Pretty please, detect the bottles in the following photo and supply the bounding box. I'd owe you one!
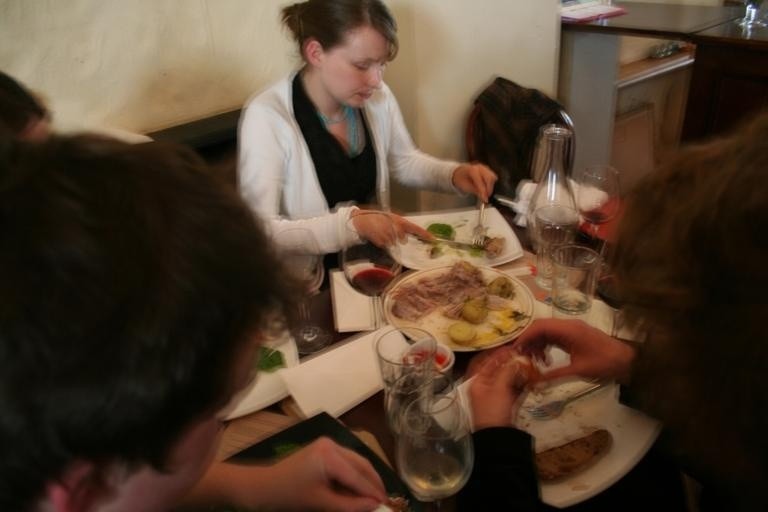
[525,127,580,253]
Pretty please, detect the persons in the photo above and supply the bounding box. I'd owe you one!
[0,125,403,512]
[442,98,768,512]
[1,72,54,143]
[235,0,499,299]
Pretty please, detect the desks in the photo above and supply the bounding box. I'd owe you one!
[560,3,765,44]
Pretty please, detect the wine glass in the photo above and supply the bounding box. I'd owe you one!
[269,228,333,356]
[376,326,475,512]
[576,163,622,238]
[342,213,402,330]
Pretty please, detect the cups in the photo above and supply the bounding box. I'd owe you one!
[534,206,603,313]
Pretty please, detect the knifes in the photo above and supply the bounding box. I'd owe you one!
[426,236,484,251]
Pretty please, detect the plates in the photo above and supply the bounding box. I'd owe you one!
[220,336,300,425]
[380,260,536,354]
[424,371,663,507]
[381,205,524,270]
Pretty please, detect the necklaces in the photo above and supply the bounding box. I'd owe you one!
[317,107,358,159]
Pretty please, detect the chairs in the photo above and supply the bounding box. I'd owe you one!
[466,75,577,208]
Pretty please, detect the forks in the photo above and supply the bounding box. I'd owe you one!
[472,199,487,247]
[527,382,611,420]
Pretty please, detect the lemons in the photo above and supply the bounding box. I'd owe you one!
[446,320,475,344]
